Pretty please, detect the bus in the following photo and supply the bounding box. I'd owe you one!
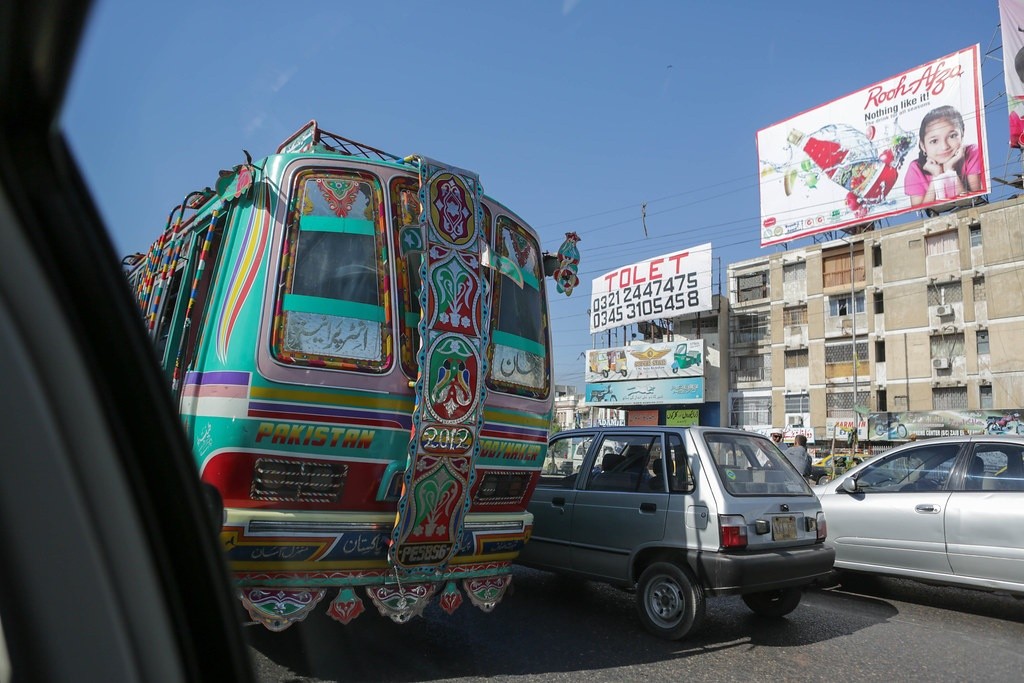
[128,126,582,606]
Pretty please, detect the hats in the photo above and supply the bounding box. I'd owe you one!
[770,428,782,434]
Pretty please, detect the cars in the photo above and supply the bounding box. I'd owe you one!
[514,426,837,643]
[815,452,888,487]
[808,436,1023,599]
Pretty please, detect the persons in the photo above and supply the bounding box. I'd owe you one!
[592,444,653,481]
[904,105,981,205]
[756,430,788,467]
[1010,46,1024,147]
[782,435,812,483]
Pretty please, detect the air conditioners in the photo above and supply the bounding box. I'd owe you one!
[789,415,801,426]
[935,304,953,318]
[932,358,949,370]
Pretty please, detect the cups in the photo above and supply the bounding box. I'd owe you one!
[934,174,955,200]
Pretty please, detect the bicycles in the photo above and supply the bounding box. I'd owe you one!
[874,415,908,438]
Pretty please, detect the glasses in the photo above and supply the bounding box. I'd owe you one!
[771,434,782,438]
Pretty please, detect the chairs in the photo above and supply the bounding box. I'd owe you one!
[653,459,676,490]
[593,453,637,489]
[981,471,1002,490]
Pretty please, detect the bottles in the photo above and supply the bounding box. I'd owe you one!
[787,130,898,204]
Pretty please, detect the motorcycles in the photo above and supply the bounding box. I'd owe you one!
[670,343,704,373]
[587,352,610,377]
[984,410,1024,434]
[607,349,629,377]
